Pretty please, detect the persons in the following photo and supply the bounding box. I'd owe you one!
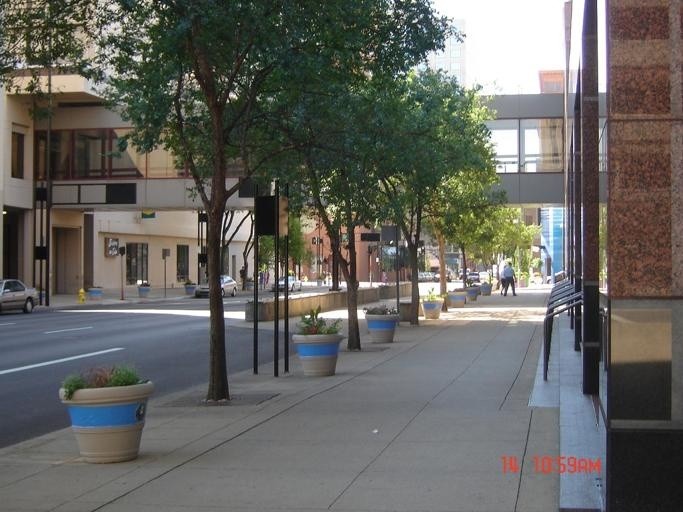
[504,263,516,296]
[501,265,507,295]
[240,266,246,280]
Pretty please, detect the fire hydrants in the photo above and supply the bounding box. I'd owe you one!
[78,288,86,304]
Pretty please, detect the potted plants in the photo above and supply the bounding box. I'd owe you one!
[291,281,494,378]
[58,363,155,464]
[87,278,199,301]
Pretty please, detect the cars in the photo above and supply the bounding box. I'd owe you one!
[195,275,238,298]
[407,270,452,282]
[272,276,302,292]
[0,279,38,314]
[458,268,491,282]
